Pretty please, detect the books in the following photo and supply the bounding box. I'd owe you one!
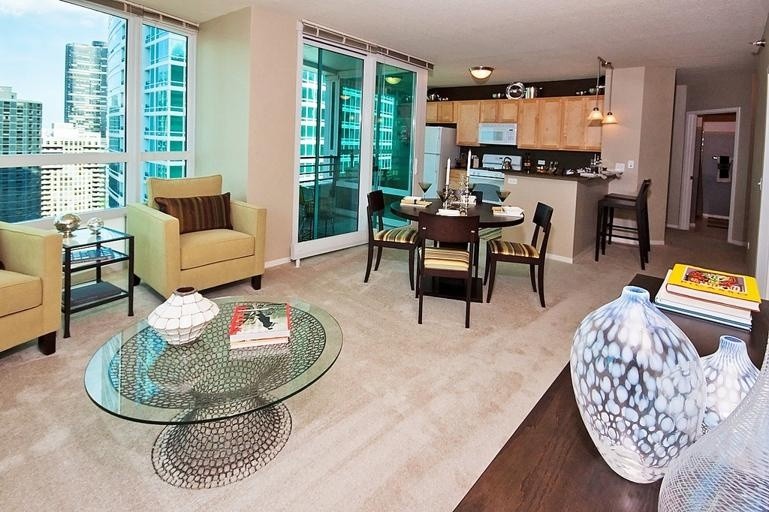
[652,262,762,331]
[491,206,525,218]
[227,302,292,349]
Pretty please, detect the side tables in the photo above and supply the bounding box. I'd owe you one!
[57,225,135,338]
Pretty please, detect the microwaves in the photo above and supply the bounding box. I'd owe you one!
[477,122,517,145]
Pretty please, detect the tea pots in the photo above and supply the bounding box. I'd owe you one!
[498,157,512,170]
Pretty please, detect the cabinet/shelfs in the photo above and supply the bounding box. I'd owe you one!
[517,97,561,150]
[426,101,453,123]
[481,99,518,123]
[561,95,604,152]
[455,100,480,147]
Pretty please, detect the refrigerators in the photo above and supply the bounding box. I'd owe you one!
[420,126,460,198]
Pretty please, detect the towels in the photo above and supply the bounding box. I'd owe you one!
[401,199,432,205]
[492,207,512,211]
[460,208,466,216]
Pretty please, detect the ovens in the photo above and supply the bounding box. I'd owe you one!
[467,176,504,206]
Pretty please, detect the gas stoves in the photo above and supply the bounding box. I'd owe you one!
[469,153,522,179]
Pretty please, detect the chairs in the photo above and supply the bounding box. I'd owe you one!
[300,185,335,239]
[483,202,553,308]
[595,183,650,271]
[416,210,480,329]
[434,187,483,278]
[604,178,650,250]
[364,190,419,290]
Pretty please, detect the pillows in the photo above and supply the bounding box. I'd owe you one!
[155,192,233,235]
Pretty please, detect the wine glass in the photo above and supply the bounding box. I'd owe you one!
[437,191,449,210]
[420,183,432,200]
[465,182,476,195]
[495,191,511,214]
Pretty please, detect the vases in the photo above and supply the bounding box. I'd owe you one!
[700,334,761,439]
[570,285,707,485]
[657,326,769,512]
[146,286,220,346]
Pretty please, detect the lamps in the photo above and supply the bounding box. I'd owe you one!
[587,57,606,120]
[601,61,618,123]
[385,77,401,85]
[341,95,350,100]
[469,66,493,79]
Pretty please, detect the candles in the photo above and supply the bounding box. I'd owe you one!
[467,149,471,176]
[446,157,451,184]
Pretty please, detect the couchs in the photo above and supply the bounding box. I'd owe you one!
[0,221,63,355]
[127,178,267,300]
